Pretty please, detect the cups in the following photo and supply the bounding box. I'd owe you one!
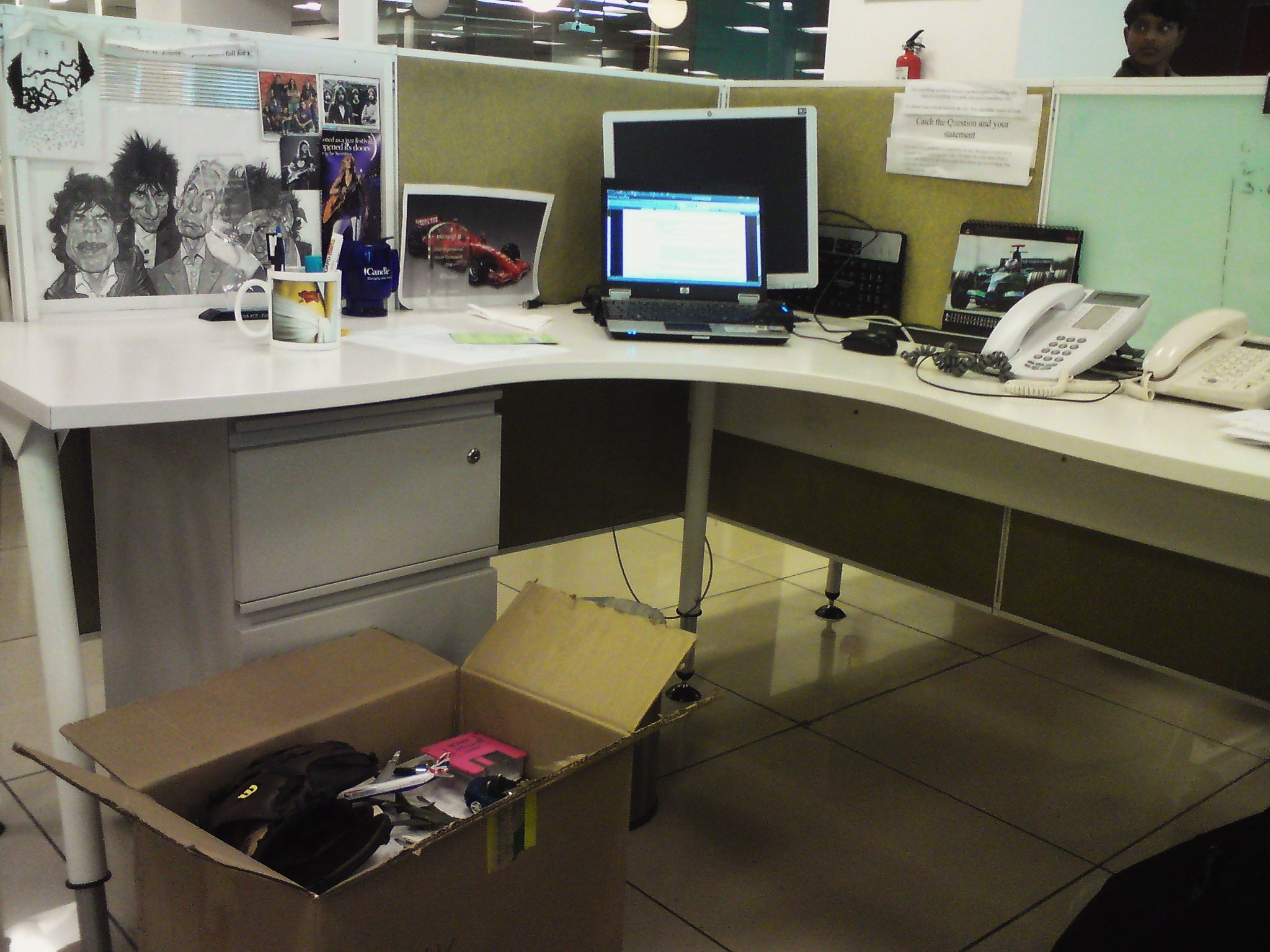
[334,242,393,318]
[235,266,342,351]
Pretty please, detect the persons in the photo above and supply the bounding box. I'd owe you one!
[288,140,314,190]
[329,154,369,241]
[262,74,319,137]
[323,85,377,126]
[1112,0,1187,78]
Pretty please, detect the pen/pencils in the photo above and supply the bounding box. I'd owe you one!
[274,225,285,271]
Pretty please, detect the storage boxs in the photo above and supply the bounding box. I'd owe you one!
[14,584,700,952]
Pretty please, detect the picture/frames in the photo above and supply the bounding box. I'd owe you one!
[257,69,321,142]
[319,74,383,135]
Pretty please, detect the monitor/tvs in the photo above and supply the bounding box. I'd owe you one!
[604,105,819,290]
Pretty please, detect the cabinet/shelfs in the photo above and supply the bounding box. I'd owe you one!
[89,385,504,712]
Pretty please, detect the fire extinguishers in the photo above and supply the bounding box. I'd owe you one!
[895,28,926,80]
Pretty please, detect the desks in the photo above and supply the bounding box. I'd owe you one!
[0,298,1269,891]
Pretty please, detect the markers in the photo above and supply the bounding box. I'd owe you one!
[324,233,343,273]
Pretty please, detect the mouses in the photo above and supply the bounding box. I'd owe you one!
[840,330,899,356]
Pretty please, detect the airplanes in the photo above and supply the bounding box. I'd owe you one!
[337,750,461,831]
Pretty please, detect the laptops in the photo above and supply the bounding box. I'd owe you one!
[600,176,792,343]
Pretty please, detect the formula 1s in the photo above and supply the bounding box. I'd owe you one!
[406,215,531,285]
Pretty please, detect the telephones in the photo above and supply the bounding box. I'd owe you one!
[976,282,1154,380]
[1139,307,1270,411]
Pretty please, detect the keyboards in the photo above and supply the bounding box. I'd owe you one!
[768,224,908,322]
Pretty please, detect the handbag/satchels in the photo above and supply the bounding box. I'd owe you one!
[189,737,393,894]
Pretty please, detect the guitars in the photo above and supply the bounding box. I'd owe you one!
[286,164,319,185]
[323,168,377,225]
